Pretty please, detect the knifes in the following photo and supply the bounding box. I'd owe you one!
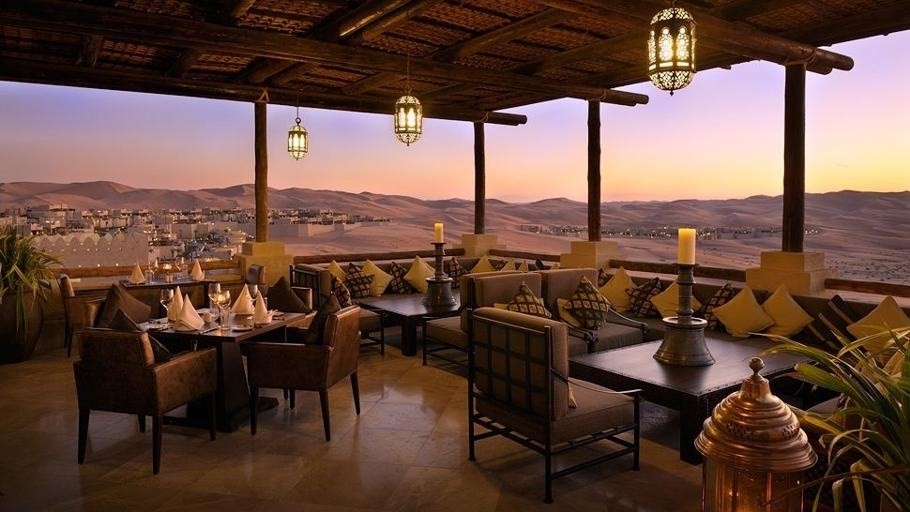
[200,325,220,334]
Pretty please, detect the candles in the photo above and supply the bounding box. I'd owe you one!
[434,222,445,242]
[678,226,697,264]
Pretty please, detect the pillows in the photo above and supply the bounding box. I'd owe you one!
[94,282,154,326]
[264,276,313,315]
[109,310,173,363]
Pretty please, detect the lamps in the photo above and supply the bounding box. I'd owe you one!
[394,44,425,147]
[286,79,309,162]
[644,0,697,97]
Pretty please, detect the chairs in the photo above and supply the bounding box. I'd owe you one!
[243,263,267,296]
[69,326,220,475]
[238,284,317,359]
[243,303,364,442]
[289,256,597,376]
[468,266,890,507]
[82,296,108,328]
[58,273,87,358]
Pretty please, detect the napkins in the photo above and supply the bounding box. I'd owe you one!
[175,293,205,332]
[168,286,184,321]
[253,288,277,324]
[190,259,205,281]
[230,282,254,313]
[128,260,146,284]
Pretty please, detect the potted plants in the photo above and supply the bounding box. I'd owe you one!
[1,220,72,364]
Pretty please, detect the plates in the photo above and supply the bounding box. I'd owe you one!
[145,323,163,329]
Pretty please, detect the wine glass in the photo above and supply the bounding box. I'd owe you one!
[160,282,267,330]
[144,256,189,283]
